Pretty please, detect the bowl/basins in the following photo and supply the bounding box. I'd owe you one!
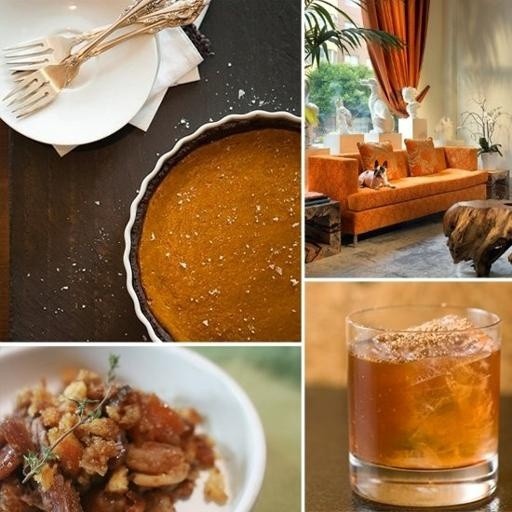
[122,110,302,343]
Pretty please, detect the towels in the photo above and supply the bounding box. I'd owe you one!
[51,0,212,158]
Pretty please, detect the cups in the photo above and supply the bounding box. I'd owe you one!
[345,303,503,511]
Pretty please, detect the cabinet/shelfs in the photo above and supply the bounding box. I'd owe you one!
[480,168,512,199]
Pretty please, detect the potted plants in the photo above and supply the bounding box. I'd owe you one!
[459,98,512,170]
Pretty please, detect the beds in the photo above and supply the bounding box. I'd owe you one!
[305,200,341,263]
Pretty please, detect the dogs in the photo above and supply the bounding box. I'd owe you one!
[357,159,396,191]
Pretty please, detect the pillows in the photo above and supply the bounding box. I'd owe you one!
[357,141,400,181]
[404,137,439,177]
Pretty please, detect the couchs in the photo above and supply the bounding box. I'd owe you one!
[308,146,489,243]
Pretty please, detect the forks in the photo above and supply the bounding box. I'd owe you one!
[1,0,211,118]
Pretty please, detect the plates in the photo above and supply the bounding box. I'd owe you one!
[1,346,268,511]
[0,0,159,146]
